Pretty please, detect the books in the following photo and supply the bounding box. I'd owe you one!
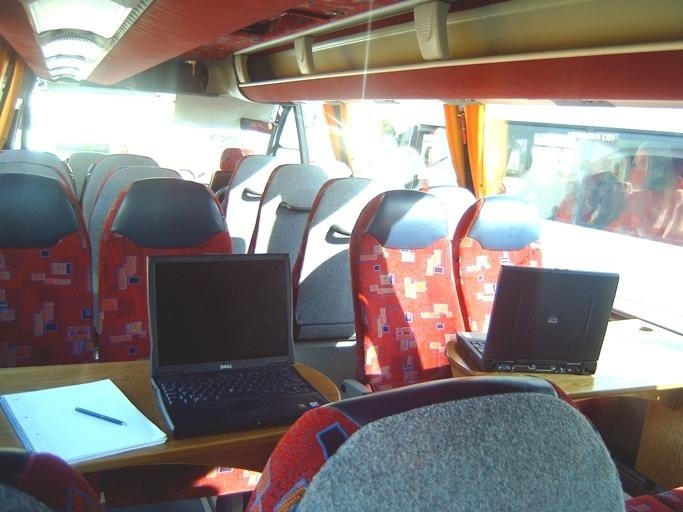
[0,377,168,467]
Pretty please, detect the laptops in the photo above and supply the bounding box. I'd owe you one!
[146,253,331,438]
[456,265,619,374]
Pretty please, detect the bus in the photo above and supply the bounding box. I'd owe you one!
[306,96,683,361]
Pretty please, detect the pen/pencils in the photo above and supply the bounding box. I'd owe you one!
[75,407,126,425]
